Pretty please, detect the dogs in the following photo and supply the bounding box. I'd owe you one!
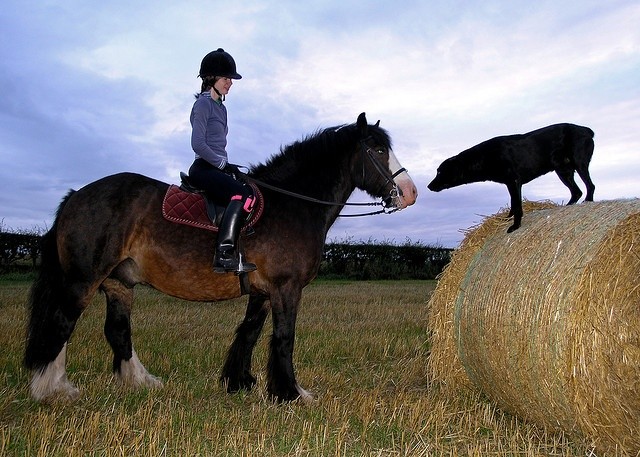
[426,120,596,233]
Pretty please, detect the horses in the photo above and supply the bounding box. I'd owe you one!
[20,111,420,411]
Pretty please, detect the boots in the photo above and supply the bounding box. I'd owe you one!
[212,198,257,273]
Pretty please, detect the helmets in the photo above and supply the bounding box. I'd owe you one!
[200,48,242,80]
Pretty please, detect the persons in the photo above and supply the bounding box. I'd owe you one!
[188,48,260,274]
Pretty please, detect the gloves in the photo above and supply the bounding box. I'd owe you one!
[224,163,239,176]
